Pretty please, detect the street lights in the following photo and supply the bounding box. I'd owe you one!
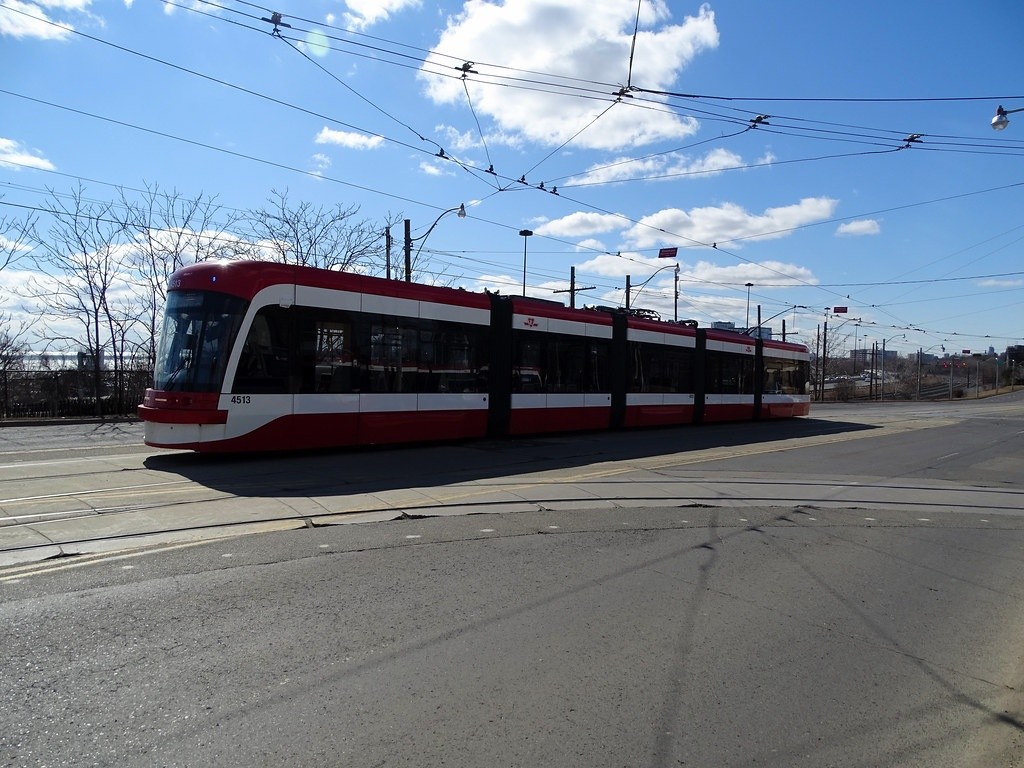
[744,304,807,338]
[401,202,467,283]
[816,318,862,402]
[915,344,947,400]
[879,334,907,401]
[947,350,1024,401]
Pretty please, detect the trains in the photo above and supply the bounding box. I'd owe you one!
[139,260,810,456]
